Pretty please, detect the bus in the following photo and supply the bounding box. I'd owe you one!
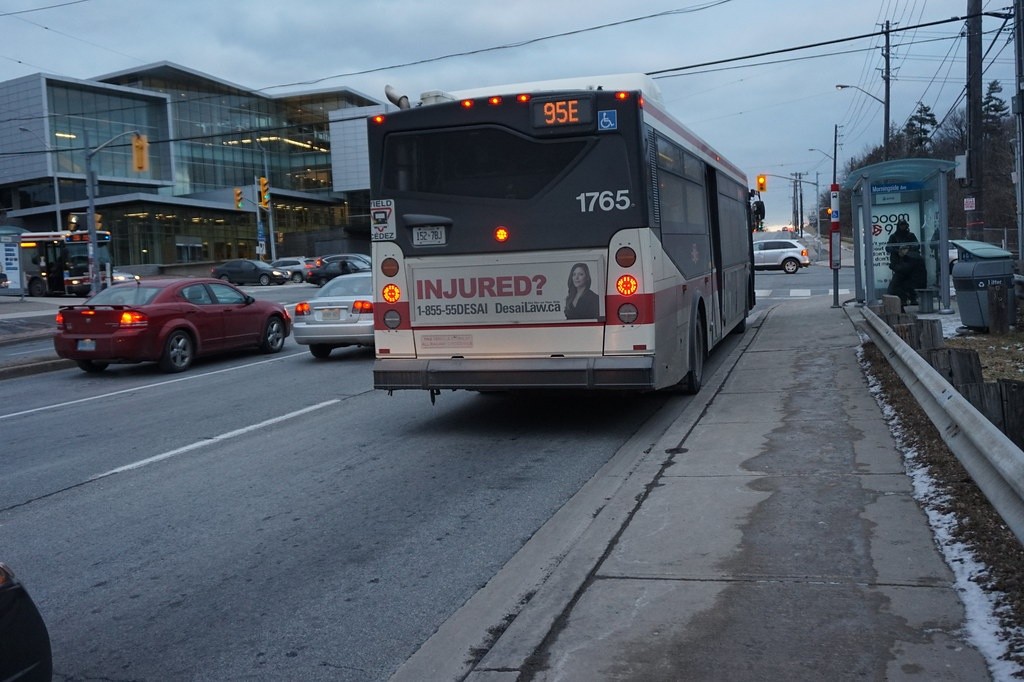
[21,230,112,296]
[366,73,766,396]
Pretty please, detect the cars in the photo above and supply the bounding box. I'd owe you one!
[111,269,139,285]
[211,258,291,285]
[946,228,969,274]
[53,274,291,373]
[292,272,375,357]
[782,227,788,231]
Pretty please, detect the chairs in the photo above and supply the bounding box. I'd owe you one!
[187,287,204,304]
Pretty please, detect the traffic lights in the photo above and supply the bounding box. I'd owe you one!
[756,175,765,191]
[234,188,243,210]
[827,208,831,219]
[95,213,103,231]
[68,212,88,232]
[260,177,270,209]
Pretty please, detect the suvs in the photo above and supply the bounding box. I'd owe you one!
[269,253,373,288]
[753,239,810,274]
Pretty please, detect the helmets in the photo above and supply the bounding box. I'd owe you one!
[896,219,909,229]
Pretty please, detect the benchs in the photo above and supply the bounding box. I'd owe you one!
[915,288,939,313]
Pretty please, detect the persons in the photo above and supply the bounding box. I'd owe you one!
[564,264,598,320]
[0,262,8,289]
[885,220,928,313]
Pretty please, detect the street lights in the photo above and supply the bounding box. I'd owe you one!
[836,19,890,161]
[809,124,844,185]
[18,127,62,232]
[235,125,276,262]
